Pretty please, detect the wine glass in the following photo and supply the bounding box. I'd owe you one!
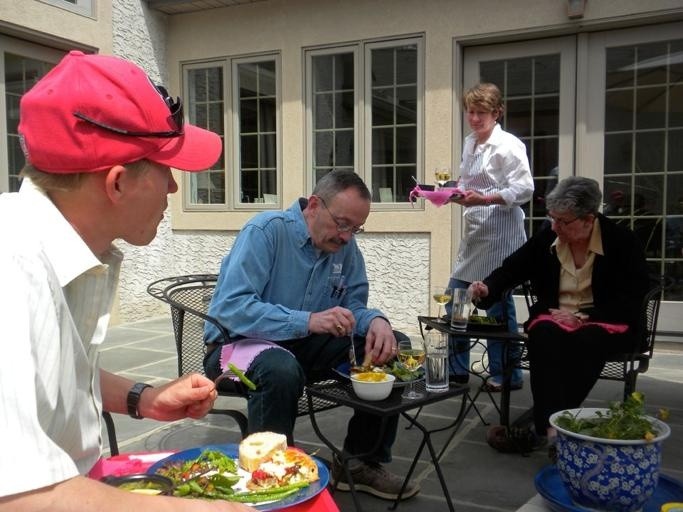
[430,287,450,325]
[397,341,425,399]
[435,168,449,187]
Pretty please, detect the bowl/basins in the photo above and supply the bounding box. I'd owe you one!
[418,184,433,190]
[440,181,457,188]
[351,372,396,401]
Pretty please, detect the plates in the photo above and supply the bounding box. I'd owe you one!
[101,473,172,495]
[442,316,506,332]
[334,361,427,388]
[145,442,329,511]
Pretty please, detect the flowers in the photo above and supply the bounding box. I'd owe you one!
[546,385,670,448]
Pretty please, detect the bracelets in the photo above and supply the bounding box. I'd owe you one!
[127,383,152,419]
[575,316,585,324]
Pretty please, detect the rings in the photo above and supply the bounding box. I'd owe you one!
[391,347,398,352]
[337,324,342,331]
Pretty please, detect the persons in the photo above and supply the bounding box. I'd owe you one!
[202,170,421,502]
[466,176,649,465]
[0,48,263,512]
[444,82,535,392]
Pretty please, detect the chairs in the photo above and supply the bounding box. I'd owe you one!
[98,401,250,499]
[143,271,352,459]
[490,264,675,437]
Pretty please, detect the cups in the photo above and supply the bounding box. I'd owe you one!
[449,287,472,333]
[424,332,448,392]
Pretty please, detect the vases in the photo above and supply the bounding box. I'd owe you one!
[544,408,672,511]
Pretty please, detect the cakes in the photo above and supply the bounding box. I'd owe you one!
[237,430,288,473]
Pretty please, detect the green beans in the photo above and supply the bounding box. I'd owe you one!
[225,363,257,392]
[212,483,310,503]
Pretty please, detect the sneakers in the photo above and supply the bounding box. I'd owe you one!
[479,379,524,393]
[486,419,551,454]
[329,448,421,500]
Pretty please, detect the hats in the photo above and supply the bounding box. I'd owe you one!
[20,50,222,172]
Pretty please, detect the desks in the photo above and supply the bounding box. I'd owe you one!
[299,364,471,511]
[398,312,529,433]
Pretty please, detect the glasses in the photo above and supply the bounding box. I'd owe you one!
[317,195,365,235]
[72,85,186,138]
[546,214,581,227]
